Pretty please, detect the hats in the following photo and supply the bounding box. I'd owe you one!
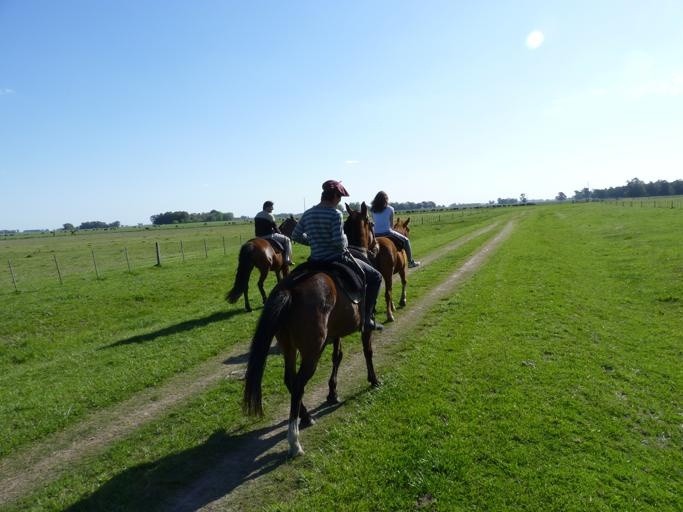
[322,180,350,197]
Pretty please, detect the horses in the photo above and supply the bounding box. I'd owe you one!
[242,200,382,459]
[226,213,299,313]
[375,216,413,323]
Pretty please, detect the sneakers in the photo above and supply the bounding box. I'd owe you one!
[369,319,384,330]
[285,261,295,265]
[408,261,420,268]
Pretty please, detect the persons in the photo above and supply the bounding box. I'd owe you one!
[253,201,296,266]
[368,191,421,268]
[291,180,384,331]
